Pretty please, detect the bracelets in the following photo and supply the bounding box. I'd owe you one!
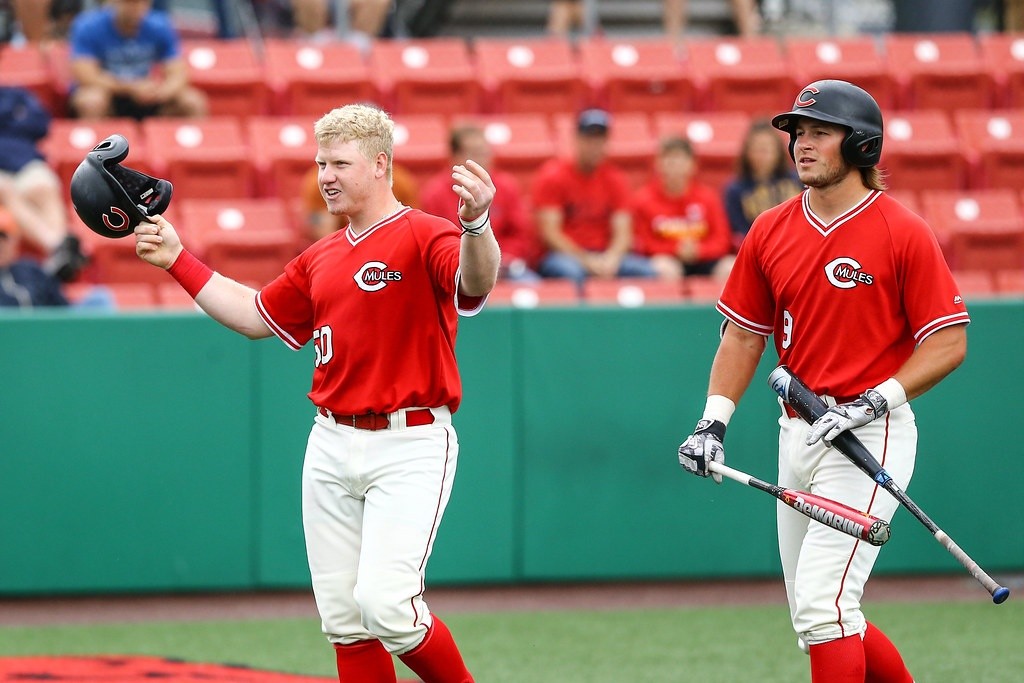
[459,213,490,236]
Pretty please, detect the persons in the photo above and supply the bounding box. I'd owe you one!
[722,116,803,255]
[419,122,538,283]
[1,0,978,46]
[133,103,502,683]
[66,0,213,124]
[629,132,739,281]
[678,79,971,683]
[0,85,88,279]
[515,108,658,280]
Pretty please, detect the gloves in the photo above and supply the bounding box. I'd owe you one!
[805,388,888,448]
[678,419,727,484]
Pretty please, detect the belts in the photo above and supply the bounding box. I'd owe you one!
[783,395,860,419]
[320,406,434,431]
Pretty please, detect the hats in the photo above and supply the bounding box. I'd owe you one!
[576,106,612,135]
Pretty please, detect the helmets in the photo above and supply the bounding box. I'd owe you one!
[771,80,883,169]
[69,134,174,239]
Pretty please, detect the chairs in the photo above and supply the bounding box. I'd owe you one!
[2,33,1024,309]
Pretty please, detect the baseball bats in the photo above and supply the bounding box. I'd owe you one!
[707,460,892,547]
[765,362,1011,606]
